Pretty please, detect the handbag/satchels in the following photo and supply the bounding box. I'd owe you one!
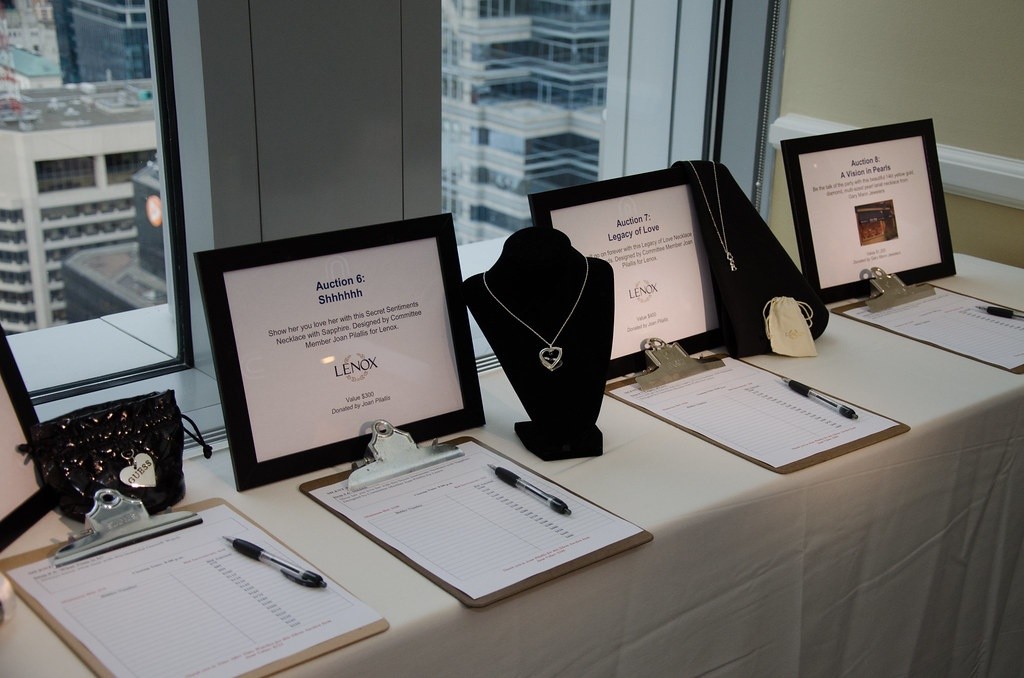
[15,388,214,524]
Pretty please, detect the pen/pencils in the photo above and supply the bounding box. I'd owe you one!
[975,305,1024,320]
[487,464,572,516]
[782,377,858,419]
[221,535,327,588]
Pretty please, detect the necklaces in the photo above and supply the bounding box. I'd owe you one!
[688,161,737,271]
[483,256,589,372]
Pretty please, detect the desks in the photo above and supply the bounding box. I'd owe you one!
[1,250,1024,677]
